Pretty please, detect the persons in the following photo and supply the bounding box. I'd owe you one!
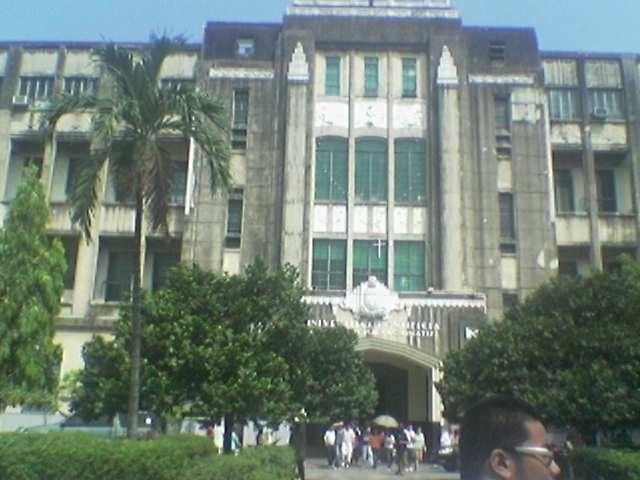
[207,425,214,440]
[323,419,427,476]
[231,429,241,456]
[459,394,561,480]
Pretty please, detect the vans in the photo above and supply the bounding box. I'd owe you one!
[16,408,160,439]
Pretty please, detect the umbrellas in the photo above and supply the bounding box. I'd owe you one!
[372,414,400,430]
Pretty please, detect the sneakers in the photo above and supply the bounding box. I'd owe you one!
[328,460,418,477]
